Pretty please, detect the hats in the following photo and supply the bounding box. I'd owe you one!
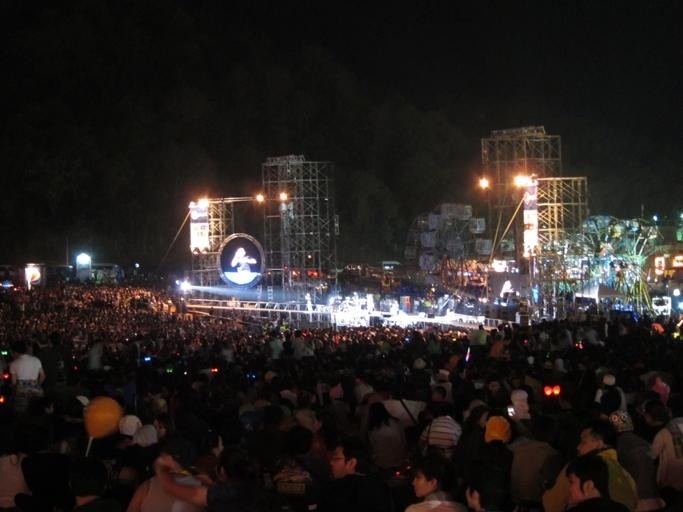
[117,414,142,437]
[129,423,160,447]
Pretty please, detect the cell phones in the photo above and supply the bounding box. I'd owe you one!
[507,406,515,418]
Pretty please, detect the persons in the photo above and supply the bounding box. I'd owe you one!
[1,267,683,510]
